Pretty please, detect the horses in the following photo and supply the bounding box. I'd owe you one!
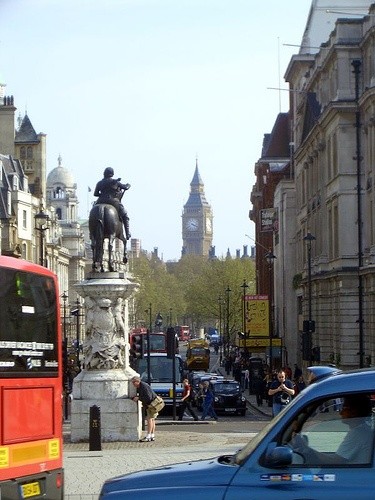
[88,188,127,272]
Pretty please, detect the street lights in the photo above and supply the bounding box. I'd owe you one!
[70,298,82,373]
[145,302,152,331]
[61,290,69,391]
[32,204,54,267]
[217,296,223,364]
[225,284,232,354]
[169,307,173,327]
[303,232,317,368]
[239,279,250,360]
[264,250,277,372]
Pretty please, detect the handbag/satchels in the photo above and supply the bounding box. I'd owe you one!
[281,393,290,404]
[149,396,166,412]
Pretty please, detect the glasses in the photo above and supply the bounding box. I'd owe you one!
[279,376,285,378]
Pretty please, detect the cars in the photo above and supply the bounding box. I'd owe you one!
[185,370,248,416]
[101,366,375,499]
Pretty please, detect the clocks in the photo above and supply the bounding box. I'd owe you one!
[186,217,200,232]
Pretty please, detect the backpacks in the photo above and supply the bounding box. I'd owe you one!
[189,386,196,399]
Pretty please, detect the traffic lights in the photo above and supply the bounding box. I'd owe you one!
[132,334,144,359]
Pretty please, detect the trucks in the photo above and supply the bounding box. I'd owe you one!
[186,338,211,371]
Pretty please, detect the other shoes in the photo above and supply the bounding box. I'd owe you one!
[139,436,151,442]
[151,436,156,441]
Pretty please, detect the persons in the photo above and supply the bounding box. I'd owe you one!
[197,381,218,420]
[93,167,132,240]
[268,371,295,418]
[131,376,159,442]
[216,339,346,411]
[287,391,374,465]
[177,379,199,421]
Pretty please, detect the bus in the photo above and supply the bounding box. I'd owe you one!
[130,328,181,355]
[0,258,67,500]
[176,325,191,341]
[136,353,188,416]
